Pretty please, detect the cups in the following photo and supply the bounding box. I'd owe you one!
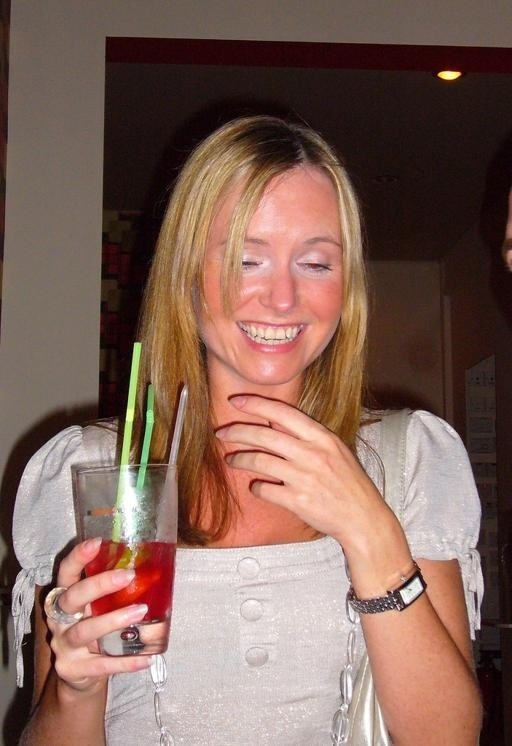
[71,464,179,659]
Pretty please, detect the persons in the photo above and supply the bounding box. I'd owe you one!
[11,112,492,745]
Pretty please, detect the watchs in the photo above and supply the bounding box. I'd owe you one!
[346,559,431,615]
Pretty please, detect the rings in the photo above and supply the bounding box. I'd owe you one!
[45,585,86,626]
